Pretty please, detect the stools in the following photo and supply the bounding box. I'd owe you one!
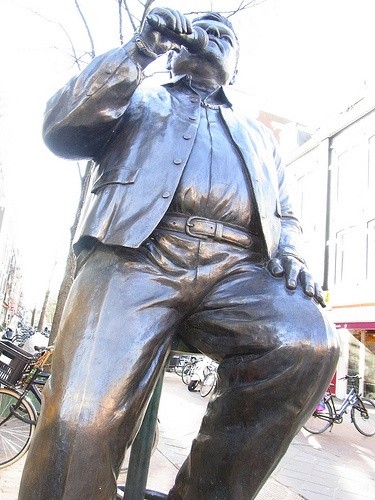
[115,332,201,500]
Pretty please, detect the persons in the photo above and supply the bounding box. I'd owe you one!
[17,5,340,500]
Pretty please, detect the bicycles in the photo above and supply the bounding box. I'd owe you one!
[302,375,375,437]
[174,355,221,397]
[0,339,160,474]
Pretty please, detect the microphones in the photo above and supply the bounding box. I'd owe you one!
[146,14,209,54]
